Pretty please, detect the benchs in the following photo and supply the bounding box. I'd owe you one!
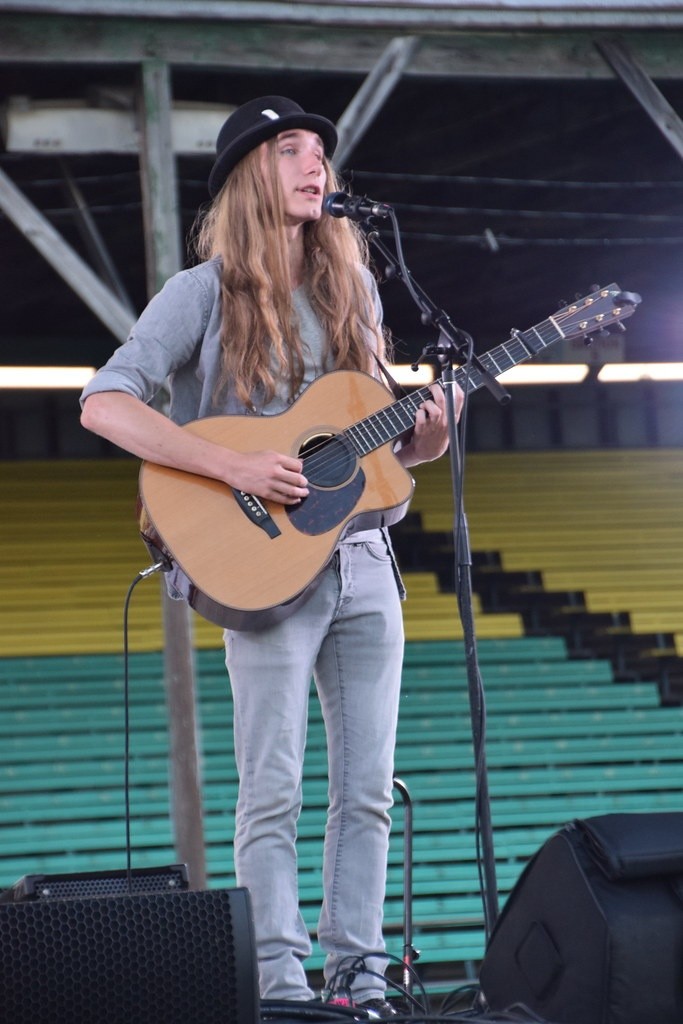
[0,442,682,1005]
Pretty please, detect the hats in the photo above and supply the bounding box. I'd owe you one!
[210,94,340,196]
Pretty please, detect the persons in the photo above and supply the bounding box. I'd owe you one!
[74,94,466,1013]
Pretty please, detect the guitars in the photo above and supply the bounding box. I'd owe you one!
[140,281,642,632]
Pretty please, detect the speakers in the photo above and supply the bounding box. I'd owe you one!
[0,886,265,1024]
[479,810,683,1024]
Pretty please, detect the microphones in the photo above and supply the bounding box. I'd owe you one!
[325,191,393,219]
